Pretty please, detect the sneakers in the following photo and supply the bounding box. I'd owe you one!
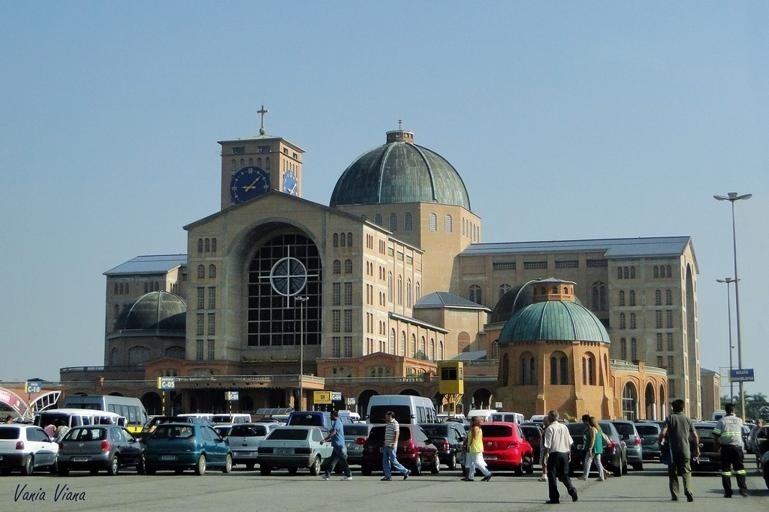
[321,470,752,505]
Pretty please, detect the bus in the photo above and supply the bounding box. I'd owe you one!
[363,394,439,424]
[63,395,149,426]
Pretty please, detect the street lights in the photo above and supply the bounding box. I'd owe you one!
[292,294,312,411]
[712,189,754,422]
[716,276,744,415]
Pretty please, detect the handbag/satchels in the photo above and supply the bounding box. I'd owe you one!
[660,418,674,466]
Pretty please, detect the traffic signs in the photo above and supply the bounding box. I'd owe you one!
[729,369,754,382]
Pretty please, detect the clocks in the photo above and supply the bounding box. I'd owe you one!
[230,166,270,206]
[282,170,299,198]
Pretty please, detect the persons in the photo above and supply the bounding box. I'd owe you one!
[712,403,751,499]
[461,410,613,505]
[749,418,764,472]
[0,415,112,443]
[658,399,699,502]
[320,410,352,481]
[380,411,411,481]
